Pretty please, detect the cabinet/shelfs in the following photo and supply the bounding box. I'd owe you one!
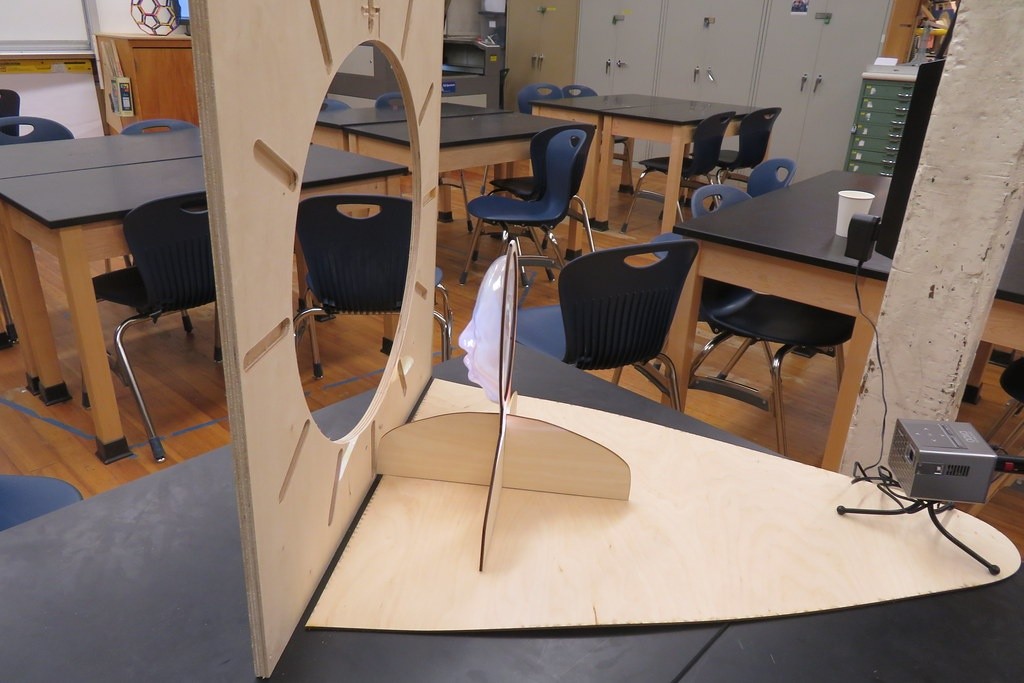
[502,0,920,190]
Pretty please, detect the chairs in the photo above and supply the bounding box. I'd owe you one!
[374,91,473,233]
[120,119,196,334]
[296,194,453,377]
[981,356,1024,453]
[459,83,882,460]
[320,99,351,114]
[0,89,20,136]
[82,190,224,462]
[0,116,74,338]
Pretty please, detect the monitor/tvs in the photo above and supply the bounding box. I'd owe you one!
[173,0,189,25]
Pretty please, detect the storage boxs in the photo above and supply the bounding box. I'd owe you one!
[110,76,134,117]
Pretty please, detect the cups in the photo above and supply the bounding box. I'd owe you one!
[835,189,875,238]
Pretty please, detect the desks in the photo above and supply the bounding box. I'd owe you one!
[593,102,764,236]
[676,479,1024,683]
[0,336,794,683]
[0,128,317,396]
[0,143,409,465]
[971,299,1024,406]
[661,170,892,471]
[312,101,513,224]
[528,94,683,229]
[342,112,597,262]
